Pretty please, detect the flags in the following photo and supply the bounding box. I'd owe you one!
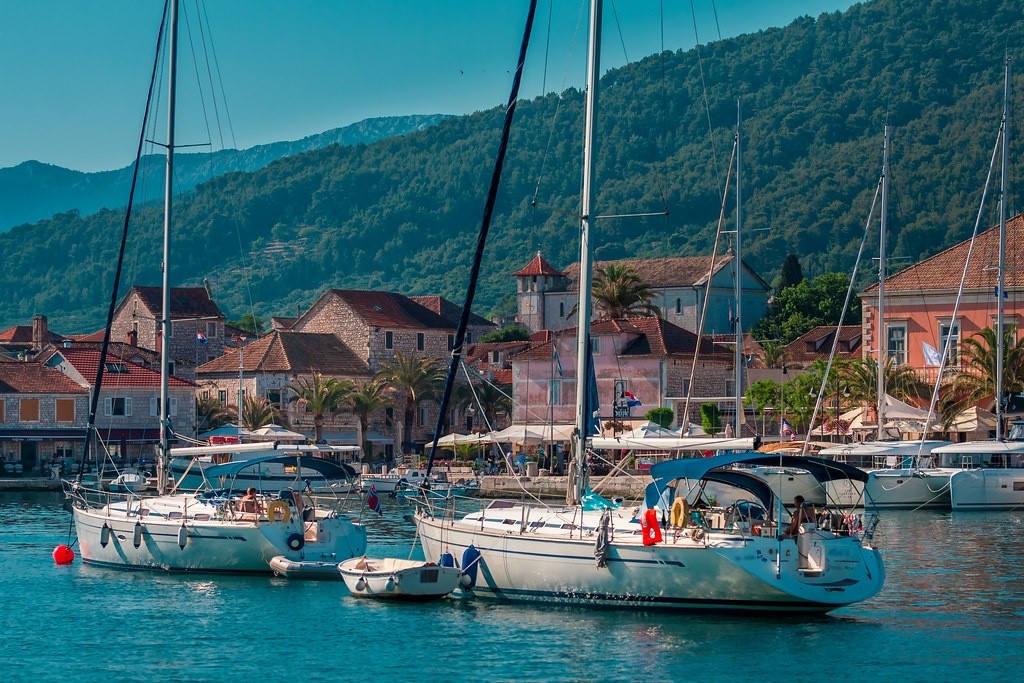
[922,341,942,366]
[779,418,796,442]
[368,485,383,517]
[553,341,563,376]
[197,334,209,344]
[624,391,641,407]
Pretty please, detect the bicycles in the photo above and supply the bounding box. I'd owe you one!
[30,463,56,481]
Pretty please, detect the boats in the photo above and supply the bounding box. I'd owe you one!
[337,556,464,601]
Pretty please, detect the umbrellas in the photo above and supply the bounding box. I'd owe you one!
[424,429,545,460]
[242,423,305,441]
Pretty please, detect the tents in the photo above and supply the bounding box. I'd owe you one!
[810,393,996,435]
[495,424,576,458]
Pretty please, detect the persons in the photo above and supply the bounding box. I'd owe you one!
[111,451,123,464]
[240,486,261,514]
[783,495,813,545]
[0,451,16,462]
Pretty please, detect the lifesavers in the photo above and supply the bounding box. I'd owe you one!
[668,496,689,528]
[267,499,291,525]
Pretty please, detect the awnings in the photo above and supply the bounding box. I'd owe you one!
[617,419,707,438]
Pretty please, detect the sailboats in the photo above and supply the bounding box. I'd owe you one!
[656,40,1023,512]
[398,0,890,614]
[60,0,385,579]
